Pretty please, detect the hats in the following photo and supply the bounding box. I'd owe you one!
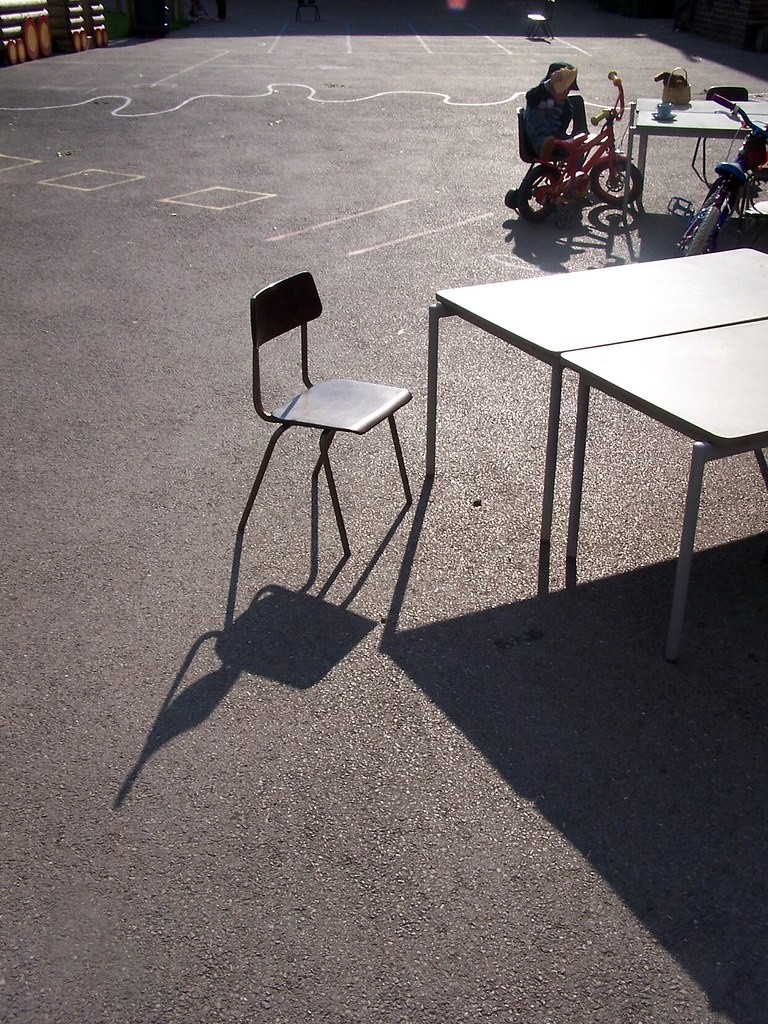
[543,62,579,91]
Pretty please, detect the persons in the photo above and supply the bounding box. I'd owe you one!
[521,61,596,206]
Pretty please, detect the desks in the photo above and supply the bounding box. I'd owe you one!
[424,248,768,544]
[559,319,768,661]
[621,98,768,218]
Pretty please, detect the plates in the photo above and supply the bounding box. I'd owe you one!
[652,113,678,120]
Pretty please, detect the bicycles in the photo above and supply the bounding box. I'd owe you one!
[668,89,768,257]
[505,71,643,222]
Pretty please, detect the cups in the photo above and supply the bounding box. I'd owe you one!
[651,103,677,121]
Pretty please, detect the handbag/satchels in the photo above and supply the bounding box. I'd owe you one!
[662,67,691,105]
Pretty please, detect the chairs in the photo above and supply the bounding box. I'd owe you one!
[566,95,589,140]
[239,270,413,557]
[526,0,555,40]
[691,85,748,177]
[516,106,564,190]
[296,0,320,22]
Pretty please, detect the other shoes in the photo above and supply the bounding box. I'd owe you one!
[578,196,594,207]
